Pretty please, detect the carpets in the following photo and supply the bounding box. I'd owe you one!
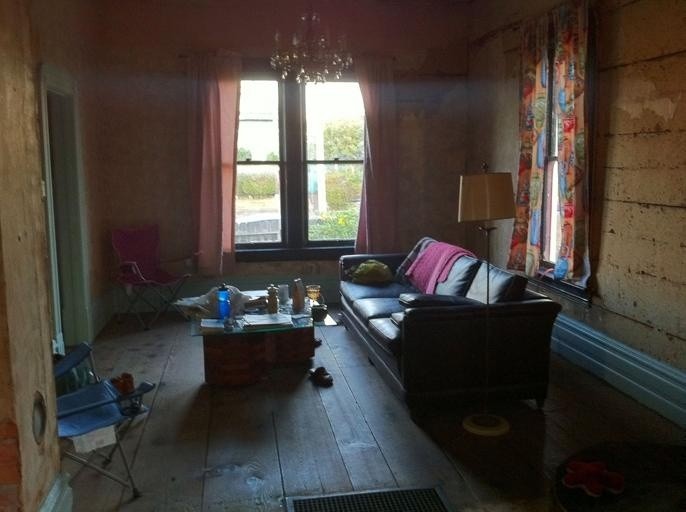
[284,485,457,512]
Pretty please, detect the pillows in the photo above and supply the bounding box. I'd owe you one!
[434,255,481,297]
[463,262,528,304]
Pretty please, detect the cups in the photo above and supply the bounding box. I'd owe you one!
[311,304,328,321]
[277,284,288,302]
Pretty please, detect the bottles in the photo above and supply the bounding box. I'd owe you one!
[215,282,231,320]
[266,283,279,314]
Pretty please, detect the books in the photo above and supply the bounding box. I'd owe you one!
[236,311,295,332]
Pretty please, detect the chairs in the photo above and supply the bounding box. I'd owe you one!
[109,223,202,331]
[54,341,156,498]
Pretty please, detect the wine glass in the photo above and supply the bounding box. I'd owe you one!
[306,284,320,306]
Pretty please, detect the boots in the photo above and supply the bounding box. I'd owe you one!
[111,373,135,394]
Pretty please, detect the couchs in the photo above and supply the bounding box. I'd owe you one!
[338,237,562,418]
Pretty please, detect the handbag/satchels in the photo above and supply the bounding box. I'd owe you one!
[352,262,393,283]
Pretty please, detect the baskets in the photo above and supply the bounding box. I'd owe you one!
[311,293,328,322]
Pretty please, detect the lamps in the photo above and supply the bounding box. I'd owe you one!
[458,159,517,437]
[270,0,353,84]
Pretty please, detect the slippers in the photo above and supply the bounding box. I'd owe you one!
[308,366,333,385]
